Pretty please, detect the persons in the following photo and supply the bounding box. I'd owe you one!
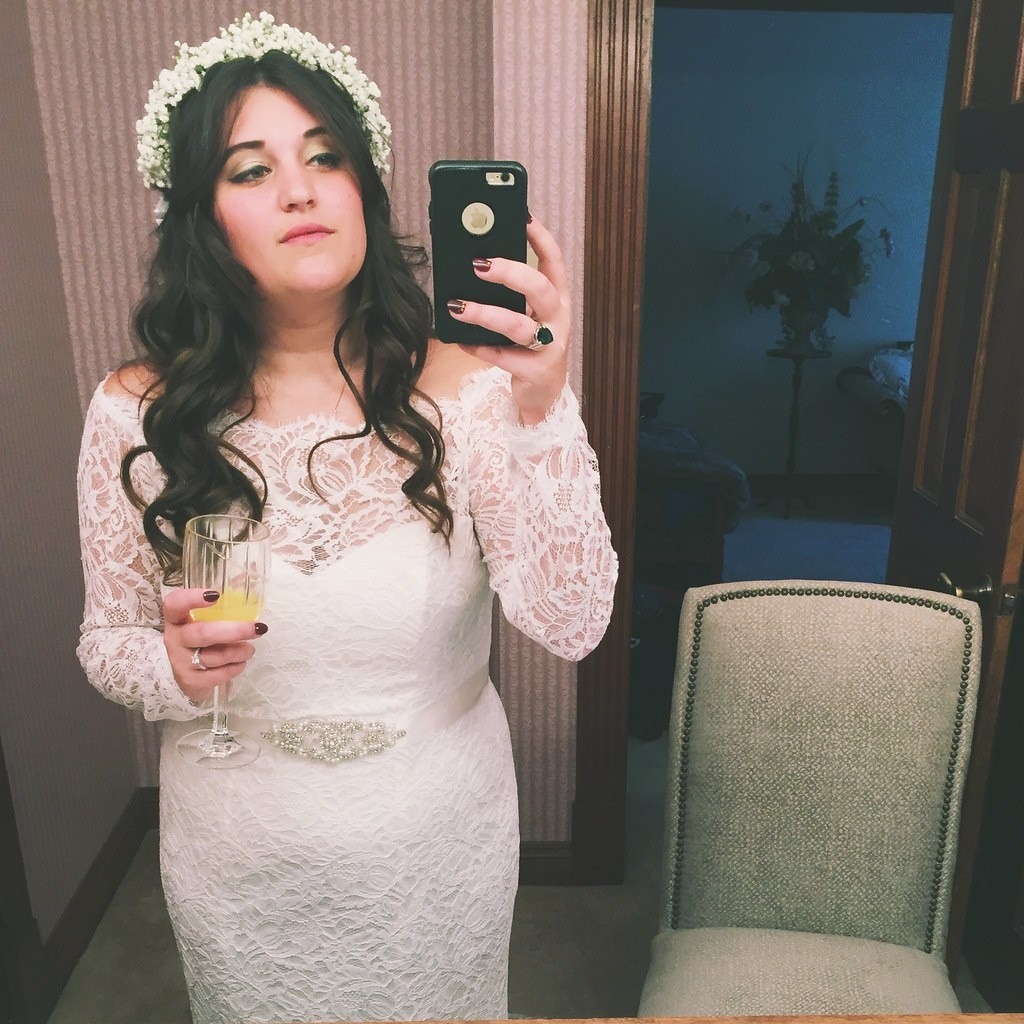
[74,10,619,1022]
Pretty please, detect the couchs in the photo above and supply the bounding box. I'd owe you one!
[627,390,754,638]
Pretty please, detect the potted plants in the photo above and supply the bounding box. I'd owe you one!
[743,147,895,519]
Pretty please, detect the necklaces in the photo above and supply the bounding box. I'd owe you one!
[261,341,355,427]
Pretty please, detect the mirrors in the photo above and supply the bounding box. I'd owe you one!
[0,0,1023,1024]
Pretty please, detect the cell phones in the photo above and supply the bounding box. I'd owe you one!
[428,160,527,344]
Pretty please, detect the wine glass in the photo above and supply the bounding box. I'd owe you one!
[178,515,272,771]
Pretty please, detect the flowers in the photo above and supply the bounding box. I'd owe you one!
[136,11,393,192]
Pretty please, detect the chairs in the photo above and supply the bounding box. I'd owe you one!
[634,576,983,1018]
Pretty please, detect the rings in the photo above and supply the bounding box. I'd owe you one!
[190,648,206,671]
[526,320,553,352]
